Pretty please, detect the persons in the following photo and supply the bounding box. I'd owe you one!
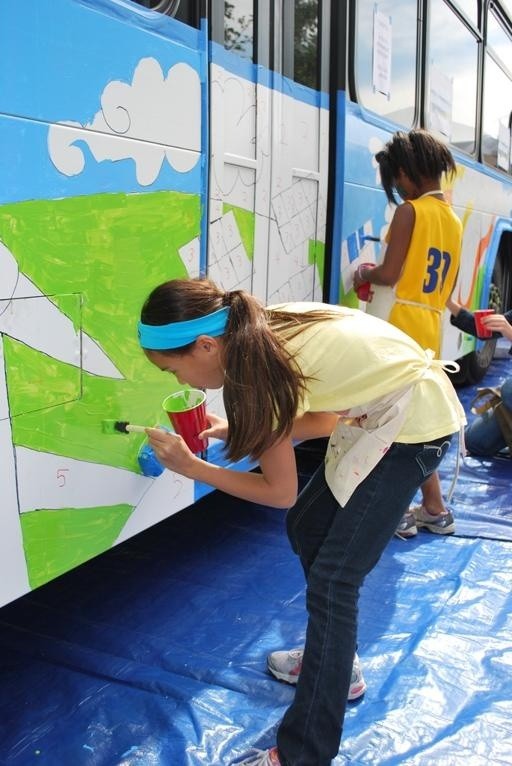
[138,277,467,766]
[354,128,462,542]
[445,293,512,457]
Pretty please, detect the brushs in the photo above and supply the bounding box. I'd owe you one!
[102,420,182,441]
[362,235,380,241]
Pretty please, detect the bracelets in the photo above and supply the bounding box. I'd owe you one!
[358,264,366,279]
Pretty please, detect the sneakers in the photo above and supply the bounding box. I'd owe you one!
[232,745,282,766]
[264,647,367,703]
[408,504,456,536]
[394,512,418,539]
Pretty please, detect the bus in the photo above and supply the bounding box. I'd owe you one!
[0,0,511,613]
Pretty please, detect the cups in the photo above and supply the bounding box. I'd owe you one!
[357,262,376,301]
[473,309,496,339]
[161,389,212,454]
